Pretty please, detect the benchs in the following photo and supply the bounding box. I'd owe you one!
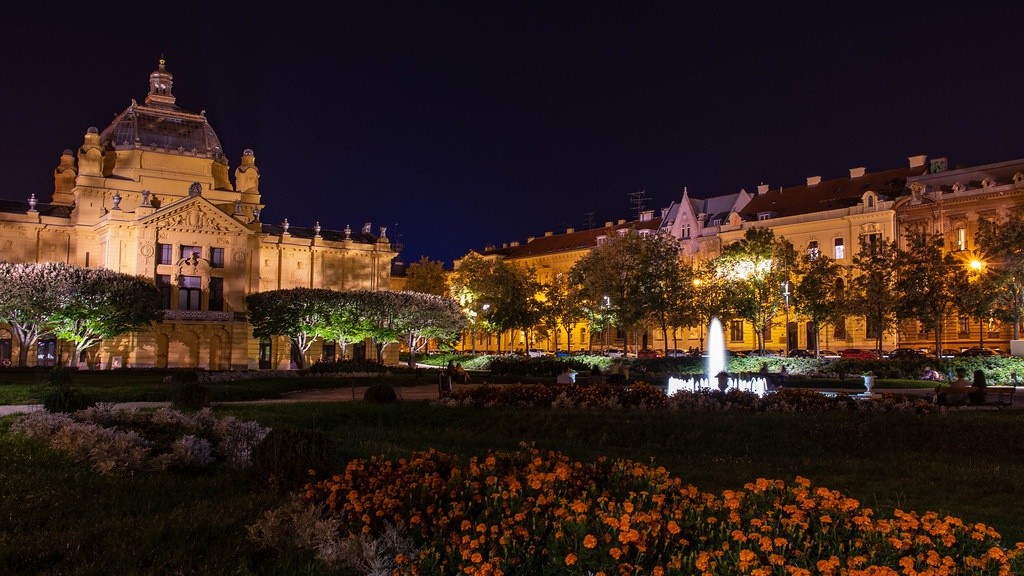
[925,385,1017,407]
[442,371,491,385]
[555,372,652,386]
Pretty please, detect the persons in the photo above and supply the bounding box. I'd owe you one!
[940,368,987,405]
[448,360,472,384]
